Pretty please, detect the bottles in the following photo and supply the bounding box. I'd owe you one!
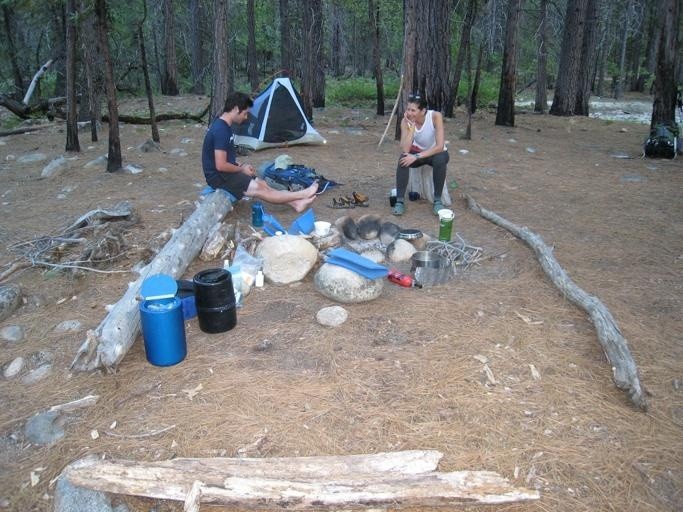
[388,270,423,290]
[256,271,264,286]
[252,200,265,226]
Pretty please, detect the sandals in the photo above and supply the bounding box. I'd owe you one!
[348,192,369,207]
[326,195,355,208]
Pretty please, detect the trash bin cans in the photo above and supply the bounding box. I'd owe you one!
[138,272,187,366]
[193,268,237,334]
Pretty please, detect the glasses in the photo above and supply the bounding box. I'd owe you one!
[409,94,422,99]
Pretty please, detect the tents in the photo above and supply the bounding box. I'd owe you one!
[224,76,327,151]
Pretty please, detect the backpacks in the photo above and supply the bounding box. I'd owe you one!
[259,161,336,194]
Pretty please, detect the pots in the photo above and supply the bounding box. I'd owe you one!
[411,250,442,268]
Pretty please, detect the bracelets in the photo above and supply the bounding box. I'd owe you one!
[414,153,420,160]
[408,127,415,132]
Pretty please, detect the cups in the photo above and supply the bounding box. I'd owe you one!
[439,209,455,241]
[314,221,331,235]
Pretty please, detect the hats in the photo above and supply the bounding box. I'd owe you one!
[274,155,293,170]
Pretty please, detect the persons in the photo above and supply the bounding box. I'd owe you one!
[201,90,319,214]
[391,93,450,218]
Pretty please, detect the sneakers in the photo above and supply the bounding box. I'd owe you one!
[394,202,404,215]
[432,202,445,215]
